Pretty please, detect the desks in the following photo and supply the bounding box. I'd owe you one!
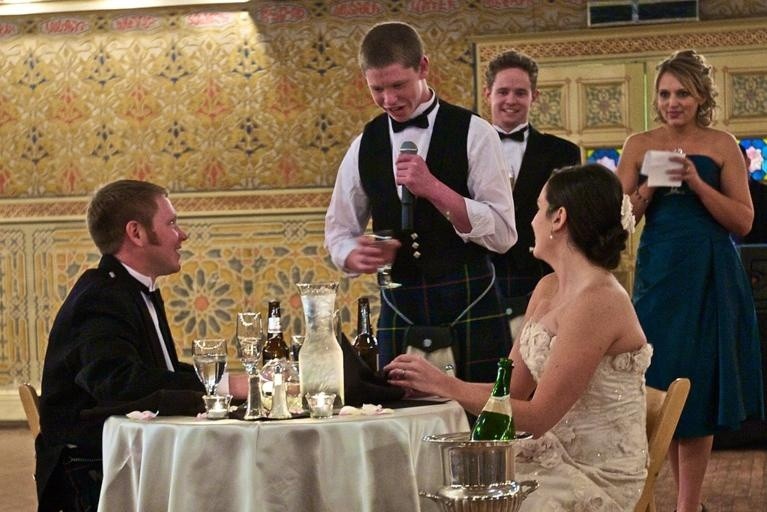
[94,394,472,512]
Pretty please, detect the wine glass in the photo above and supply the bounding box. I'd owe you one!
[192,338,228,395]
[361,227,404,290]
[236,311,264,410]
[665,148,687,197]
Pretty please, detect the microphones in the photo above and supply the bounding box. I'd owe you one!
[399,139,418,231]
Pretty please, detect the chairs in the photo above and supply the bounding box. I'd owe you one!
[631,376,691,512]
[18,383,43,439]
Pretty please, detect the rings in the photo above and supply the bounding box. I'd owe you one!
[400,370,405,378]
[685,166,689,174]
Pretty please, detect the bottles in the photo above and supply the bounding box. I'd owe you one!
[244,365,268,420]
[353,297,380,373]
[266,364,294,420]
[258,358,300,411]
[469,355,520,443]
[297,281,346,415]
[261,299,289,368]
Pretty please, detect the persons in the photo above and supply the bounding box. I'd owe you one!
[36,180,299,512]
[484,52,581,337]
[615,50,765,512]
[325,23,518,380]
[731,143,767,244]
[382,165,654,512]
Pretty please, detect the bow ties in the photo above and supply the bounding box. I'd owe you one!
[391,117,429,132]
[149,287,162,301]
[495,131,525,143]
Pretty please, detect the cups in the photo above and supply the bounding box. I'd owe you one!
[505,163,515,190]
[303,391,336,420]
[202,394,233,420]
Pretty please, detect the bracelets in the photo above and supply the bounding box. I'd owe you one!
[635,184,650,205]
